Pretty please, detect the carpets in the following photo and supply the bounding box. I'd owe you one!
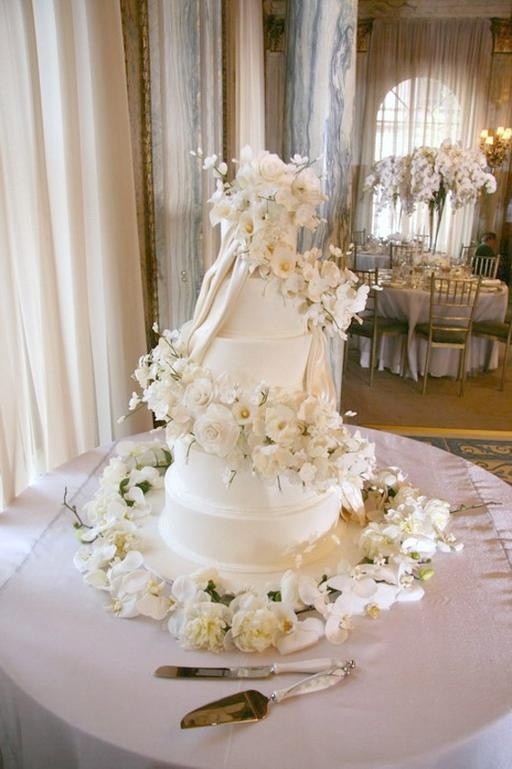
[369,426,510,492]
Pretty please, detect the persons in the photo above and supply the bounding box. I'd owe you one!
[473,232,500,278]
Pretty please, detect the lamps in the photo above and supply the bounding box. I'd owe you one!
[479,122,512,166]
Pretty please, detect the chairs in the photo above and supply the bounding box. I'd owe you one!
[340,227,512,397]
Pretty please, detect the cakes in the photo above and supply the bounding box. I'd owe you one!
[156,180,347,588]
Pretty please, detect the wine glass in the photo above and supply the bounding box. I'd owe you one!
[359,236,502,291]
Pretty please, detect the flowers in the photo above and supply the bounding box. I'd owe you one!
[360,136,497,256]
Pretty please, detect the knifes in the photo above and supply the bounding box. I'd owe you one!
[151,656,356,680]
[178,666,351,731]
